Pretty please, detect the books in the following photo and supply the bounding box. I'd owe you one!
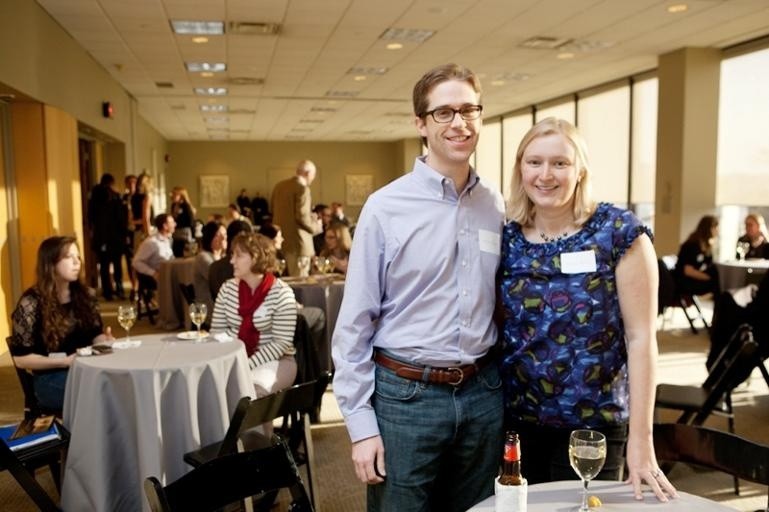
[0,413,63,453]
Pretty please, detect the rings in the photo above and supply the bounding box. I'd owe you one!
[653,469,662,478]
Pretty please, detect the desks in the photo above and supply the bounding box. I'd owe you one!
[60,332,260,511]
[713,258,767,282]
[467,476,734,510]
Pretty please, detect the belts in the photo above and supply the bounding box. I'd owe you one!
[375,353,489,384]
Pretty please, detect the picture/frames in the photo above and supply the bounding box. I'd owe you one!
[197,173,230,209]
[265,166,321,213]
[344,173,374,207]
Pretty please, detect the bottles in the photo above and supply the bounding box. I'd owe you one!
[494,429,528,511]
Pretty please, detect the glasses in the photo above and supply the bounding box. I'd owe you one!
[424,105,483,124]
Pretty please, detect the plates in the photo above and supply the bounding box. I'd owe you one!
[178,331,209,339]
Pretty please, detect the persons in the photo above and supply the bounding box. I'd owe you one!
[494,116,680,503]
[329,60,509,512]
[10,234,116,416]
[735,211,769,260]
[85,158,354,333]
[677,214,721,303]
[206,228,299,454]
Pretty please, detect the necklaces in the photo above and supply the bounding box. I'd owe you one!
[531,215,574,243]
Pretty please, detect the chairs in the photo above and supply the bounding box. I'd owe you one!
[281,313,324,428]
[5,334,70,420]
[659,253,707,333]
[622,421,768,510]
[653,321,762,497]
[714,287,768,403]
[132,247,352,338]
[182,372,331,509]
[143,433,315,509]
[1,418,73,511]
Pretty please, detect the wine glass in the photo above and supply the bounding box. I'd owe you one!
[736,242,750,263]
[277,256,336,281]
[189,303,207,342]
[118,305,136,345]
[567,428,607,512]
